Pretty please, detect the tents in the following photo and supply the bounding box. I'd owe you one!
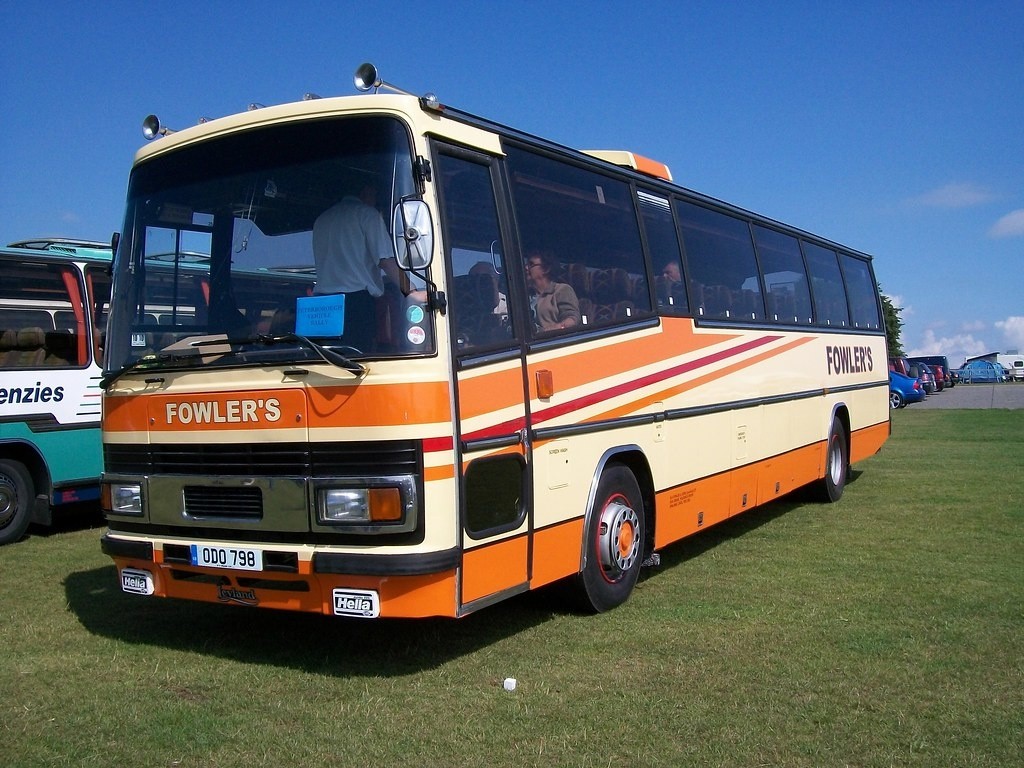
[958,361,1011,384]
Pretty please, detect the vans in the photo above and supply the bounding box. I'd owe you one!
[890,355,960,396]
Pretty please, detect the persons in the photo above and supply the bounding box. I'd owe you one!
[309,158,433,362]
[458,259,516,349]
[659,259,691,284]
[503,248,582,343]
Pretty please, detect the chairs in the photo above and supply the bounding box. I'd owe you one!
[452,257,876,351]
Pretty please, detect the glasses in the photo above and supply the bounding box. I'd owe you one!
[525,263,543,267]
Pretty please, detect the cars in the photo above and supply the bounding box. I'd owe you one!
[888,368,925,409]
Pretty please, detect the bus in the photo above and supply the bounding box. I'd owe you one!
[0,238,320,551]
[95,64,889,625]
